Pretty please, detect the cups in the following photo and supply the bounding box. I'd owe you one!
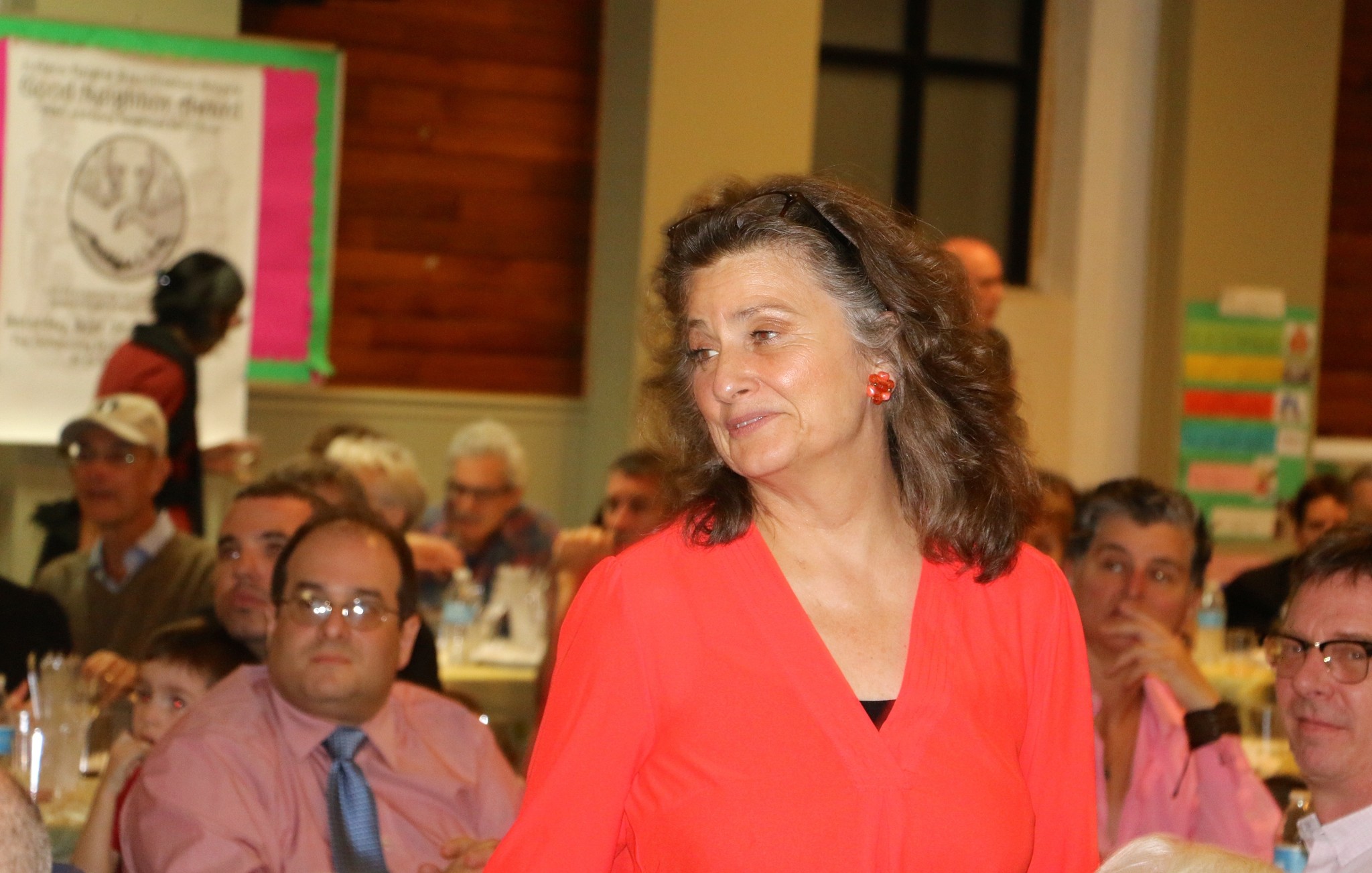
[10,653,94,861]
[510,574,549,645]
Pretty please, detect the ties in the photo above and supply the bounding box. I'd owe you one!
[321,727,390,873]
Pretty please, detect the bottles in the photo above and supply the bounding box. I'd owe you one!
[436,566,482,672]
[1272,790,1311,873]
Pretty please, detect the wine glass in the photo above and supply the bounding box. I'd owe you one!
[1204,629,1280,766]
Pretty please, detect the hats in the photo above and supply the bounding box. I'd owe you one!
[61,392,169,458]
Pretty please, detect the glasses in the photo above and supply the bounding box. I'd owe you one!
[226,313,242,328]
[446,477,510,498]
[278,588,404,632]
[1261,631,1372,684]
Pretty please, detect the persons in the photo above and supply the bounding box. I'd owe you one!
[482,172,1103,873]
[1,248,689,873]
[945,231,1372,873]
